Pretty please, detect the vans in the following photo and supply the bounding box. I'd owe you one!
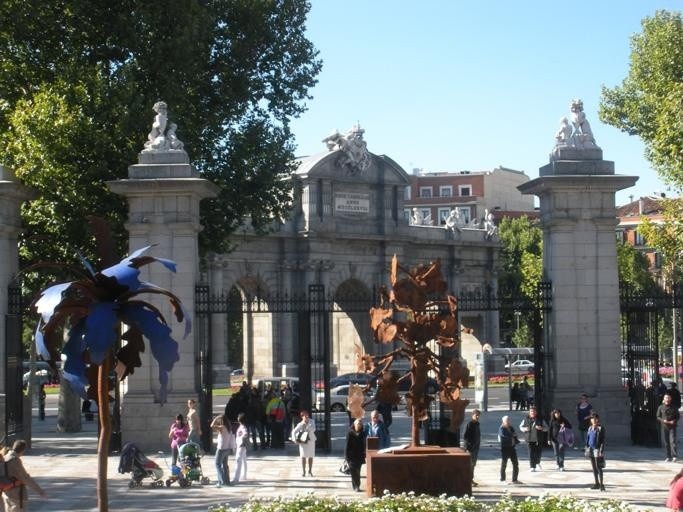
[258,375,317,408]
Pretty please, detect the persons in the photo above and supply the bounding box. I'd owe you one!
[465,410,481,487]
[365,410,390,449]
[291,410,316,479]
[168,123,185,149]
[573,393,593,451]
[585,412,605,490]
[0,446,9,512]
[519,405,550,472]
[81,399,94,422]
[628,379,681,410]
[547,409,573,472]
[665,467,683,512]
[2,439,48,512]
[556,117,573,140]
[656,393,680,463]
[168,381,297,484]
[148,102,168,140]
[409,202,495,240]
[499,415,524,484]
[511,375,535,410]
[38,385,47,421]
[344,418,366,492]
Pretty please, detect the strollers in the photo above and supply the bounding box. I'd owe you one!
[174,439,210,486]
[118,443,166,488]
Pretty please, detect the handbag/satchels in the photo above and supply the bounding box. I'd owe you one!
[597,455,606,469]
[559,429,575,447]
[339,463,354,475]
[287,431,309,444]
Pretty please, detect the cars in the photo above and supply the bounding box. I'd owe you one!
[229,369,243,383]
[309,361,439,416]
[504,359,535,372]
[621,363,683,397]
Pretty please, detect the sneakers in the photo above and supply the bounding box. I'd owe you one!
[591,484,605,491]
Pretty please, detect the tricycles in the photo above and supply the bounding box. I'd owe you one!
[157,450,192,488]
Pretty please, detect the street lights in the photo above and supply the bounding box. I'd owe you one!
[513,311,523,359]
[647,297,656,366]
